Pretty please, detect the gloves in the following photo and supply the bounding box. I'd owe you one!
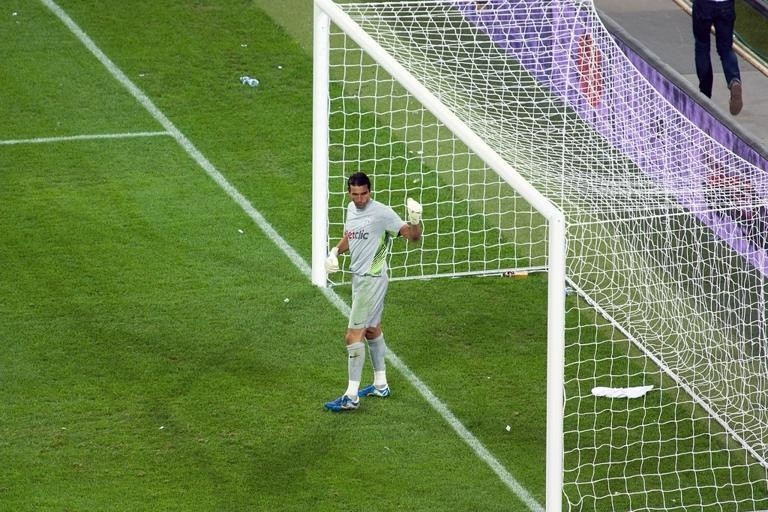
[407,197,424,226]
[324,246,341,274]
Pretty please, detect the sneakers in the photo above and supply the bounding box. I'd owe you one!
[324,393,361,412]
[728,81,744,116]
[358,384,391,398]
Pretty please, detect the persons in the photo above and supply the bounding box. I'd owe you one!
[323,171,423,412]
[690,0,743,116]
